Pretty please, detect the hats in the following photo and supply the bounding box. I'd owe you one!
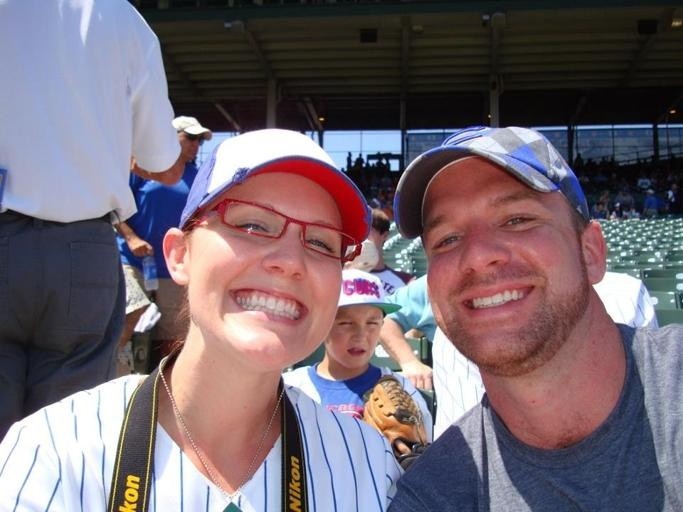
[392,125,590,238]
[179,128,373,246]
[172,115,212,140]
[337,269,402,314]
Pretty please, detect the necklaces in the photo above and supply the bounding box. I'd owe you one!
[151,358,287,492]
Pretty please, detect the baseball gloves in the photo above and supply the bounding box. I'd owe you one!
[362,377,428,458]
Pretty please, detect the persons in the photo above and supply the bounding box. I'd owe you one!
[567,146,682,222]
[430,236,652,438]
[2,2,184,426]
[340,151,430,338]
[274,270,433,466]
[116,116,213,377]
[2,126,403,512]
[379,122,682,511]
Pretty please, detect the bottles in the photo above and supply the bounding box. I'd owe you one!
[140,251,159,293]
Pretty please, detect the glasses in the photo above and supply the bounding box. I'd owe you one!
[184,133,204,145]
[181,198,362,262]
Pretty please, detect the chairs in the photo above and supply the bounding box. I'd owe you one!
[372,214,682,429]
